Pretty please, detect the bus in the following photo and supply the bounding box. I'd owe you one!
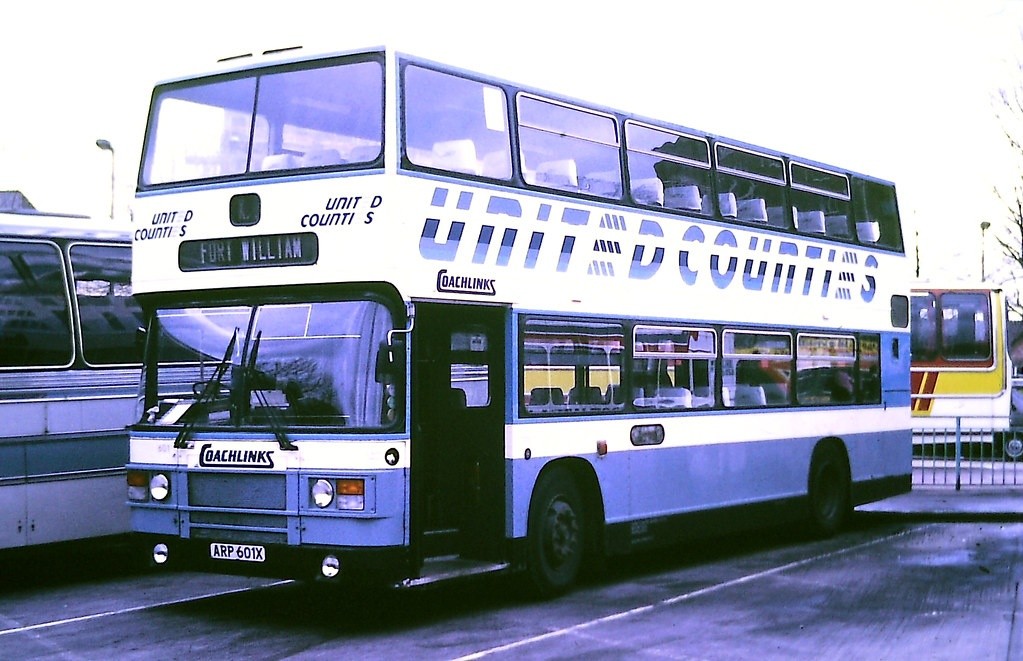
[1,190,216,555]
[907,285,1013,449]
[123,42,916,603]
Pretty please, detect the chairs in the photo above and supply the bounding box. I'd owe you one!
[452,387,467,408]
[692,386,730,407]
[664,185,703,211]
[568,387,601,408]
[528,387,565,411]
[433,139,484,176]
[826,215,848,235]
[483,149,526,182]
[798,210,826,236]
[652,388,692,409]
[734,386,766,406]
[536,158,578,187]
[586,170,620,197]
[347,145,381,161]
[737,198,769,222]
[302,148,341,167]
[606,385,644,408]
[767,205,798,229]
[631,178,664,206]
[261,154,298,171]
[702,192,738,217]
[760,382,788,403]
[856,221,881,242]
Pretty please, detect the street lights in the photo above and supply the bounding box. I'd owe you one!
[979,222,994,285]
[94,139,117,225]
